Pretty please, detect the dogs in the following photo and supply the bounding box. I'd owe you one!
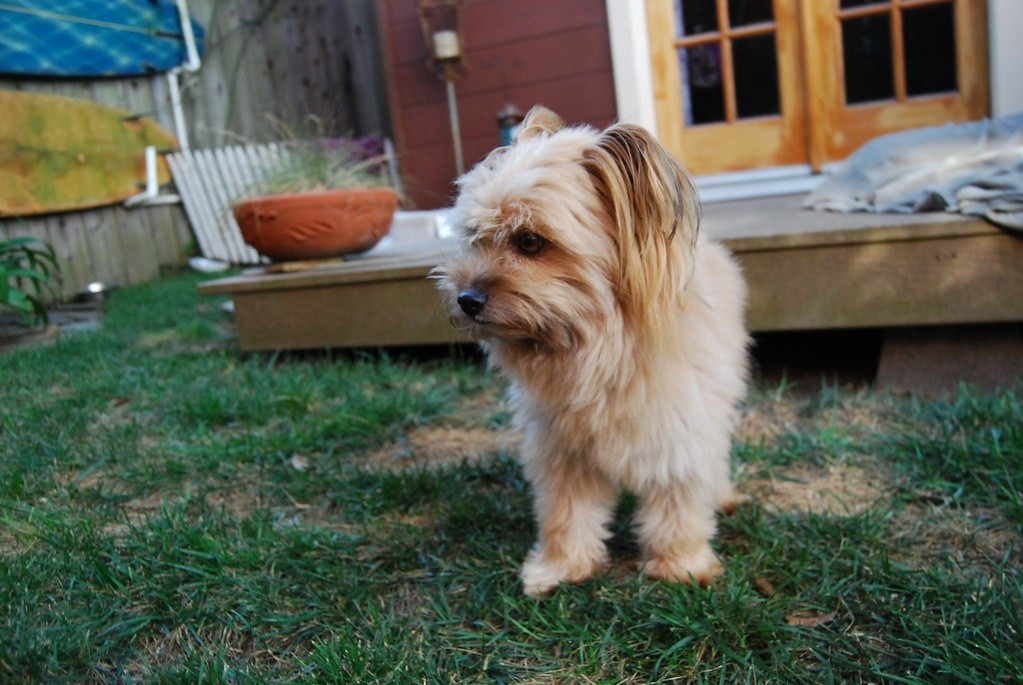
[426,102,758,604]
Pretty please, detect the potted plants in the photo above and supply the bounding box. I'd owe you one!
[198,109,434,262]
